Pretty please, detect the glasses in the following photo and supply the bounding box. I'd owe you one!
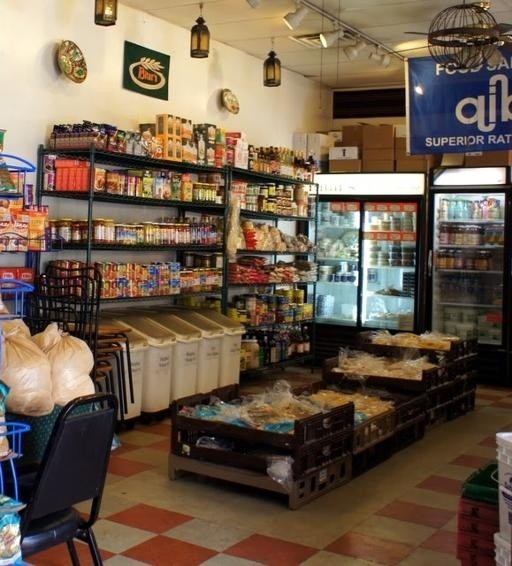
[262,37,281,86]
[191,2,211,58]
[95,0,117,26]
[282,0,406,69]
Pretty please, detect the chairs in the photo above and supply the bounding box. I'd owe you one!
[5,392,117,566]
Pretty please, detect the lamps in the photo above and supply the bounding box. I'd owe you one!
[282,0,406,69]
[262,37,281,86]
[95,0,117,26]
[191,2,211,58]
[5,392,117,566]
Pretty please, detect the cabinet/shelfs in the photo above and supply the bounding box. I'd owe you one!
[34,142,512,434]
[231,326,315,363]
[434,252,498,275]
[229,132,317,180]
[48,260,222,297]
[56,160,223,202]
[57,116,229,164]
[440,192,499,219]
[436,222,506,246]
[226,291,318,322]
[48,212,223,247]
[437,278,503,304]
[231,174,315,215]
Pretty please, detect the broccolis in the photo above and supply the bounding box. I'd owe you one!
[427,165,508,355]
[315,174,360,366]
[360,173,425,334]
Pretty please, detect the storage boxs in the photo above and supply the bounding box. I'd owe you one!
[292,121,511,173]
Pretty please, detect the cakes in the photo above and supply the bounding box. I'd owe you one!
[180,267,223,293]
[256,182,295,216]
[193,183,218,203]
[49,217,192,246]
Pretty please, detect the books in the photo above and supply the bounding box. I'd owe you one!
[292,121,511,173]
